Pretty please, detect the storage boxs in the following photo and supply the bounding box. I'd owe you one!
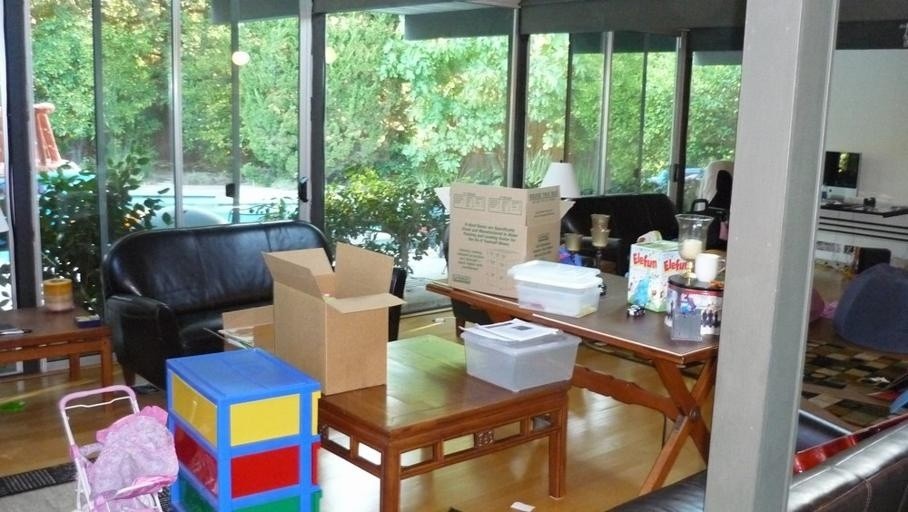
[261,241,408,397]
[509,259,603,319]
[432,180,561,299]
[221,305,274,354]
[459,318,583,393]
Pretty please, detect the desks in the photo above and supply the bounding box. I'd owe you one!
[816,203,908,265]
[318,333,573,512]
[425,269,720,498]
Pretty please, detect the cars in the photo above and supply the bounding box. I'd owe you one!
[135,201,300,228]
[645,167,702,189]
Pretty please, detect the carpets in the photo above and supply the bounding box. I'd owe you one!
[0,456,174,512]
[576,335,908,432]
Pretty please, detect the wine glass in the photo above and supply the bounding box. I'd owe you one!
[674,213,714,279]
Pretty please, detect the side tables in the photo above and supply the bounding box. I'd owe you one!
[0,303,114,414]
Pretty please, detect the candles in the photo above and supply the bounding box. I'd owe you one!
[679,239,704,262]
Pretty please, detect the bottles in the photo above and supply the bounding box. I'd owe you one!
[822,183,832,203]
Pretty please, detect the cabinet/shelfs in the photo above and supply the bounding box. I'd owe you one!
[165,346,323,512]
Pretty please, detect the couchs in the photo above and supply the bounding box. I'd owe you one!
[605,410,908,512]
[561,193,680,277]
[99,219,408,393]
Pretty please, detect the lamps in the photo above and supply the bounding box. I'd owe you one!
[539,159,583,199]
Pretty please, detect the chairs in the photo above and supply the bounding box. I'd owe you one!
[680,169,732,250]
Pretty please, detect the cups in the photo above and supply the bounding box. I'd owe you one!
[564,212,610,251]
[695,252,728,283]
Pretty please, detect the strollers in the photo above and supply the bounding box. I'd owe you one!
[55,381,179,511]
